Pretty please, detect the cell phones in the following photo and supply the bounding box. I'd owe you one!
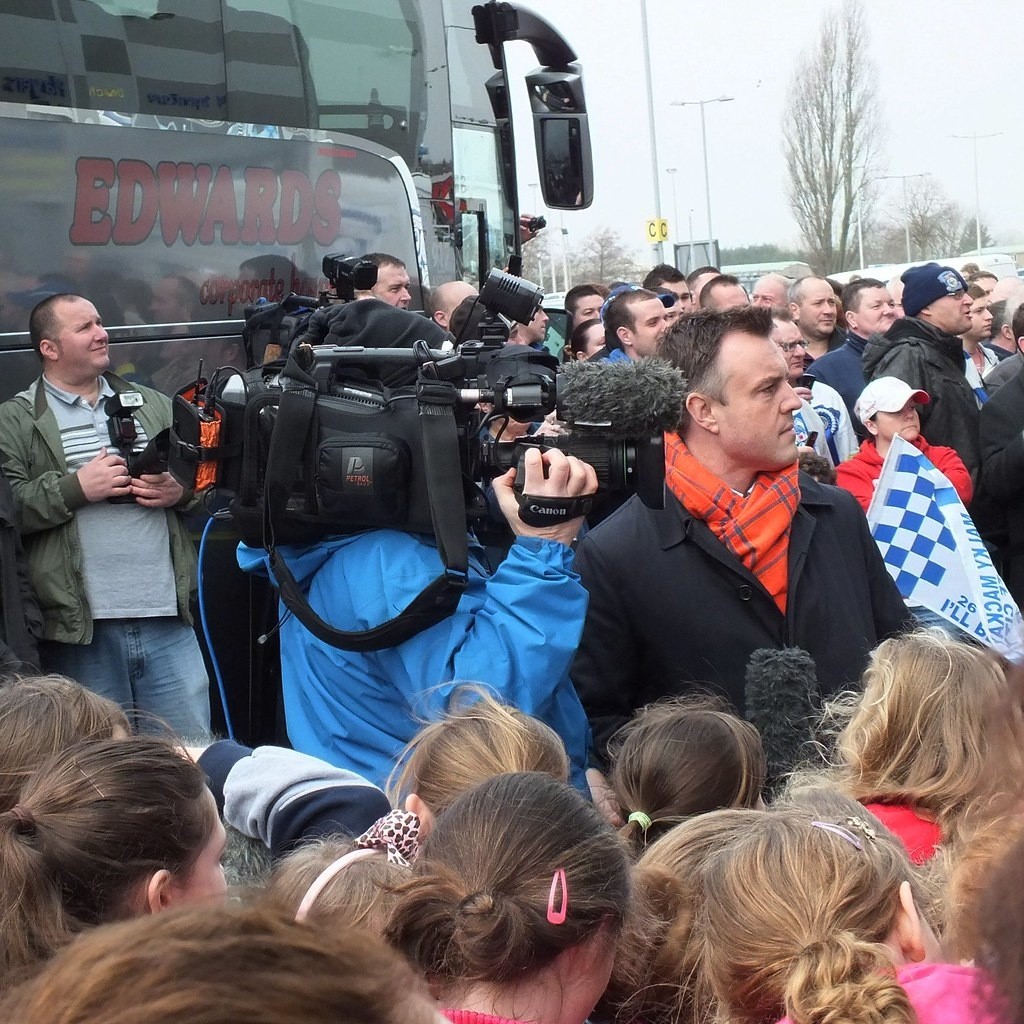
[799,373,816,391]
[805,431,819,448]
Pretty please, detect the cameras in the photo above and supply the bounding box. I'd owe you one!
[527,216,546,233]
[101,388,173,504]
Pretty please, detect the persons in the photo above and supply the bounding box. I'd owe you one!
[481,214,1024,827]
[0,246,320,396]
[0,294,215,742]
[0,629,1024,1024]
[429,281,478,330]
[237,294,596,802]
[361,254,412,310]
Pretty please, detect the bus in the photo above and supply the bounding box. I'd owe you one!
[0,0,594,402]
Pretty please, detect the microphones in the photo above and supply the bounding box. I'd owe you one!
[552,354,690,439]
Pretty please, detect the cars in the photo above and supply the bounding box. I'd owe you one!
[540,291,571,363]
[722,244,1024,300]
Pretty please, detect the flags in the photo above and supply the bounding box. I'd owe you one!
[870,435,1024,664]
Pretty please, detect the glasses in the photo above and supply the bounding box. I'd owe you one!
[779,340,808,350]
[946,291,965,299]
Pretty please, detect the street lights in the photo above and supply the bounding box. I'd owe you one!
[667,167,682,243]
[528,183,544,287]
[951,132,1004,257]
[667,95,734,267]
[875,171,932,263]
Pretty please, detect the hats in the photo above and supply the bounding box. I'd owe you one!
[301,300,458,388]
[599,285,675,328]
[901,262,970,315]
[859,375,929,425]
